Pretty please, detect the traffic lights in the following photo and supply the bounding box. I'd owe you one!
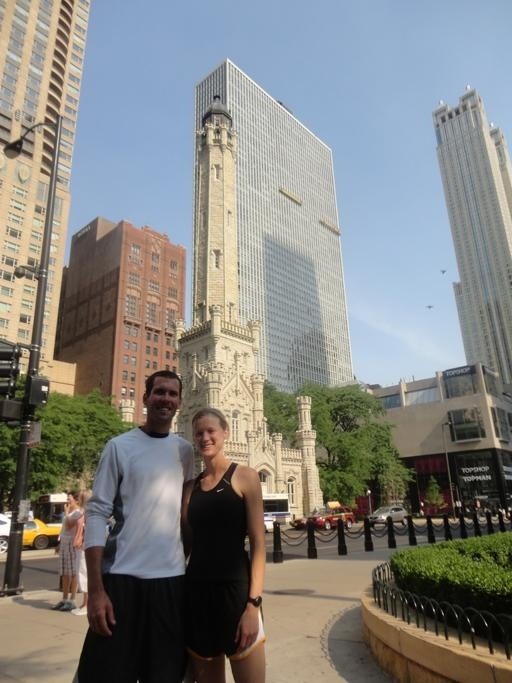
[0,336,22,398]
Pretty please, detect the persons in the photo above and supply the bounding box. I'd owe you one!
[70,487,93,615]
[179,407,267,682]
[71,369,196,683]
[51,489,84,611]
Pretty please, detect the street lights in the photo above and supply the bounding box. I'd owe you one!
[366,488,374,514]
[0,109,68,596]
[440,420,458,519]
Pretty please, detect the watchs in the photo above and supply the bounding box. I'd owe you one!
[247,594,263,607]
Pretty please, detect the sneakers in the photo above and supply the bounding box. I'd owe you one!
[53,602,88,615]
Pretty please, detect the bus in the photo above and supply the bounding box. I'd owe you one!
[35,489,69,526]
[262,491,294,535]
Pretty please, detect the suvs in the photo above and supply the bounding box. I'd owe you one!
[305,501,354,530]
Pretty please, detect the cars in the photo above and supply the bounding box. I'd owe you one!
[22,512,62,550]
[288,516,307,529]
[505,492,512,501]
[0,512,13,554]
[365,504,411,525]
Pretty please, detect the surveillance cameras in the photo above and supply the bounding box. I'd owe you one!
[14,267,25,278]
